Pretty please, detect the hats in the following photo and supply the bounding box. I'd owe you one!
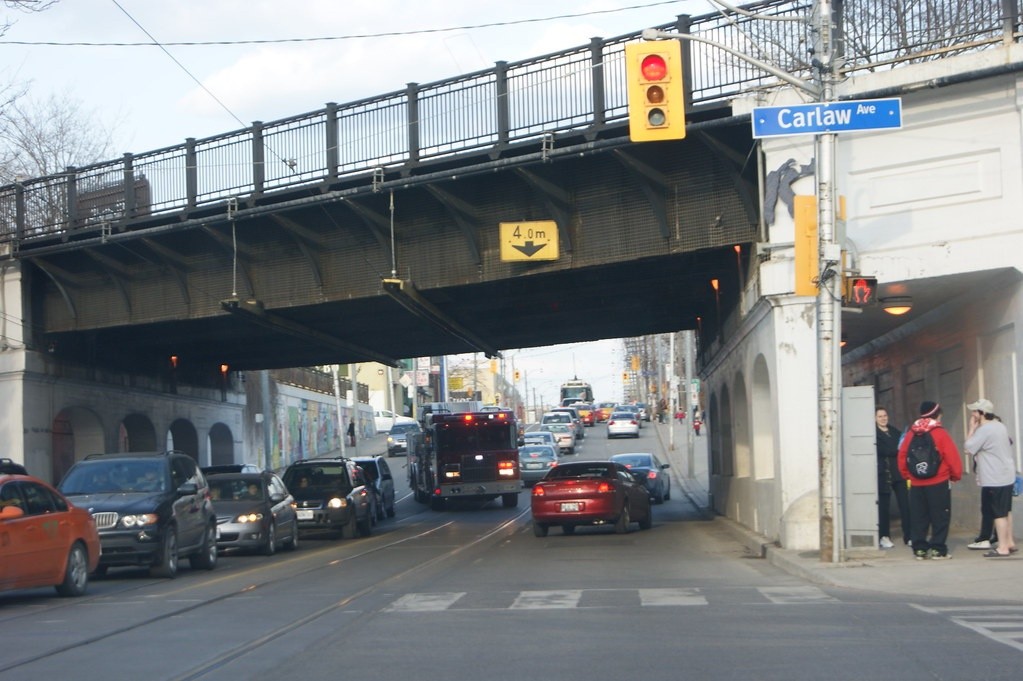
[966,399,993,415]
[920,401,939,417]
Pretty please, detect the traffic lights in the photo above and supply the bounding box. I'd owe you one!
[623,372,628,383]
[625,39,686,142]
[515,370,520,382]
[845,277,878,307]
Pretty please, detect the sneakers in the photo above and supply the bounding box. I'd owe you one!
[932,549,952,559]
[916,550,926,559]
[880,536,894,549]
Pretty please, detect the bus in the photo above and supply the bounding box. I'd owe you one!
[560,380,595,407]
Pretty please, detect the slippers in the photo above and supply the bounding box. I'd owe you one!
[995,543,1018,553]
[983,546,1009,558]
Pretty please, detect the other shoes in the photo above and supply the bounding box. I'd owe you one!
[967,540,992,550]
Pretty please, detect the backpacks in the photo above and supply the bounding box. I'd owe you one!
[906,426,943,479]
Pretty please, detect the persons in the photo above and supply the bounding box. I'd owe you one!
[694,417,703,436]
[897,401,962,560]
[657,399,670,424]
[875,406,912,548]
[94,469,110,489]
[676,408,685,424]
[298,476,308,487]
[248,484,257,496]
[963,399,1018,558]
[347,418,355,447]
[135,467,162,491]
[211,488,220,499]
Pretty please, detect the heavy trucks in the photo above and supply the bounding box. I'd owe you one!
[406,402,524,511]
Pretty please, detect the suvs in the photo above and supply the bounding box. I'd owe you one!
[386,421,424,457]
[56,450,217,578]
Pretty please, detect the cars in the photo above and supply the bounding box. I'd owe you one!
[373,409,422,432]
[518,445,563,485]
[607,411,640,438]
[199,464,299,555]
[602,453,671,504]
[524,397,596,458]
[0,457,102,596]
[281,456,395,540]
[531,460,652,538]
[596,401,650,429]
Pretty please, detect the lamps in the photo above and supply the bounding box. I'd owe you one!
[880,295,915,315]
